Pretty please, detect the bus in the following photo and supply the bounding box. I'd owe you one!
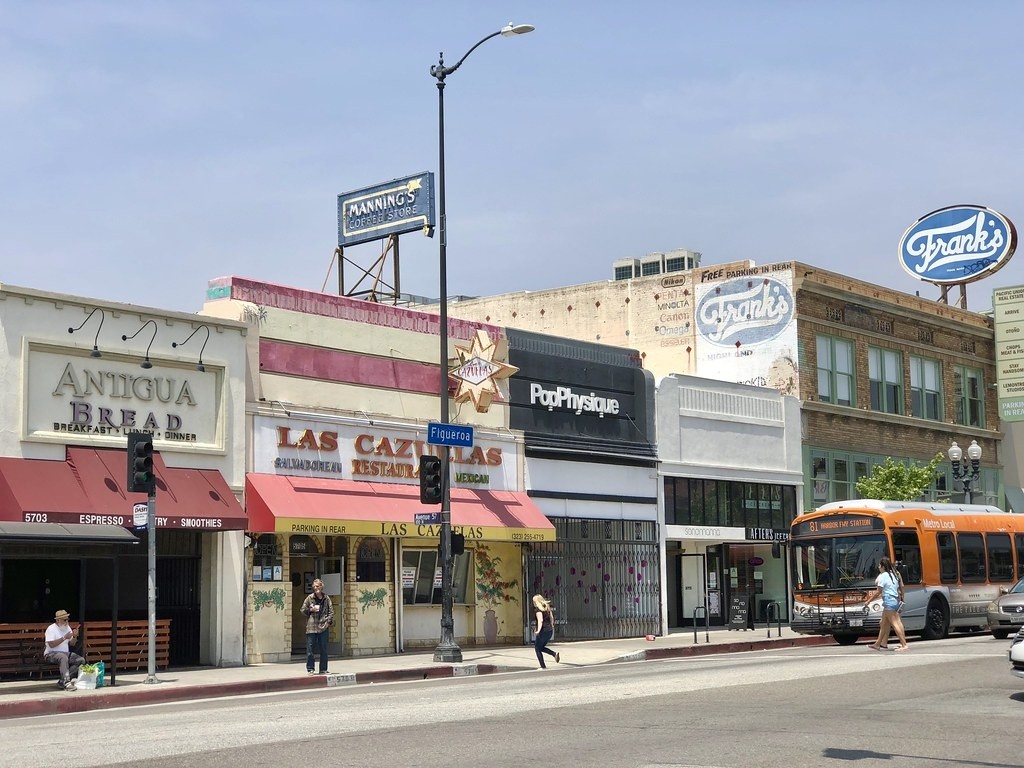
[772,499,1024,646]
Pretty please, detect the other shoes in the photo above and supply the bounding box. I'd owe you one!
[555,652,560,662]
[536,667,546,671]
[867,644,879,652]
[320,671,331,676]
[57,681,66,690]
[64,683,77,692]
[308,670,314,676]
[895,644,908,652]
[879,643,888,649]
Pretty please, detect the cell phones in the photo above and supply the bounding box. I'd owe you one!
[75,623,80,630]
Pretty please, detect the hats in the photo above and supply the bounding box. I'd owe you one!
[54,610,70,620]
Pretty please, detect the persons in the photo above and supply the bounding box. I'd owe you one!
[865,558,909,652]
[300,579,334,676]
[533,594,559,670]
[44,611,85,691]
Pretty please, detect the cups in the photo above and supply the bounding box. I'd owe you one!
[314,605,320,611]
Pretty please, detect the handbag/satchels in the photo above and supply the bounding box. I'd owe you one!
[74,662,104,690]
[896,600,905,614]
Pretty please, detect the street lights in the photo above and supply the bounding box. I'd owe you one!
[947,440,982,506]
[429,22,538,663]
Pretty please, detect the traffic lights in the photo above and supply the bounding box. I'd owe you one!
[425,460,442,500]
[133,441,153,485]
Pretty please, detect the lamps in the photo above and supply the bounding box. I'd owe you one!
[172,324,210,372]
[354,409,373,427]
[270,400,290,417]
[68,307,104,357]
[122,319,158,369]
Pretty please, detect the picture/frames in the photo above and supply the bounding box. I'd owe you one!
[304,572,315,594]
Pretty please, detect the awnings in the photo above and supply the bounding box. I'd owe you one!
[245,474,557,542]
[0,445,248,533]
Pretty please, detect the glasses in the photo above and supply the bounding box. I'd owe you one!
[63,618,70,621]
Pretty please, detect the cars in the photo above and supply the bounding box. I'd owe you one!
[1007,625,1024,680]
[986,577,1024,638]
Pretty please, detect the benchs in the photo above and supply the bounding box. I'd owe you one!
[15,637,61,680]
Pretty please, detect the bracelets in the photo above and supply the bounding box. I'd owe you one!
[62,636,66,640]
